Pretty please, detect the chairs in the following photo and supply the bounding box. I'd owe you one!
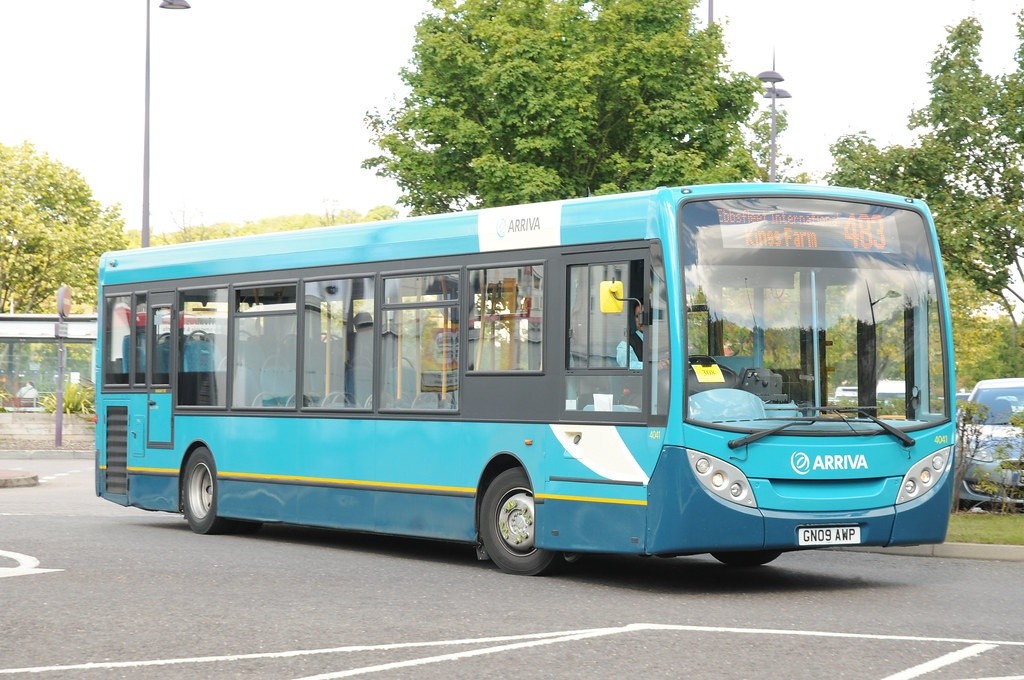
[992,398,1012,418]
[123,329,455,410]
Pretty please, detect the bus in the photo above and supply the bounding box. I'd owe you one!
[92,181,956,576]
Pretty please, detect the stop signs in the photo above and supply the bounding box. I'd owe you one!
[57,288,71,318]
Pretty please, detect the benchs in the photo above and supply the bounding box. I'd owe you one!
[2,398,34,406]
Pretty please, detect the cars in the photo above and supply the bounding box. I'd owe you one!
[957,378,1024,510]
[835,387,859,401]
[877,379,905,400]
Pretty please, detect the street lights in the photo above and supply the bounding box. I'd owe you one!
[142,0,192,247]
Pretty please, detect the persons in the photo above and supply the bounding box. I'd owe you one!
[616,297,670,371]
[16,382,38,407]
[353,312,373,332]
